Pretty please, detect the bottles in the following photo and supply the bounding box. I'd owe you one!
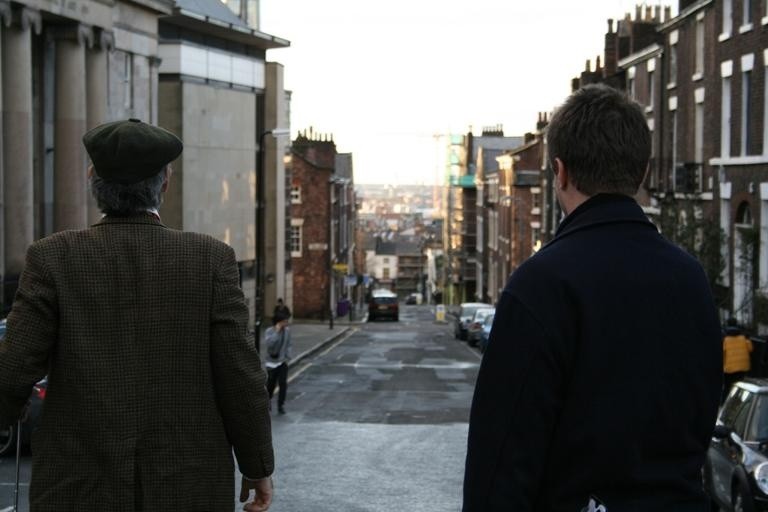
[81,118,183,184]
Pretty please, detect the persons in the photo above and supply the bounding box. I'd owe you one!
[720,318,755,407]
[1,116,276,511]
[261,312,294,415]
[461,82,727,511]
[273,298,291,324]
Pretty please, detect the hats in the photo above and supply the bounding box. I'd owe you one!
[451,302,497,355]
[701,373,768,512]
[368,292,398,321]
[0,317,49,456]
